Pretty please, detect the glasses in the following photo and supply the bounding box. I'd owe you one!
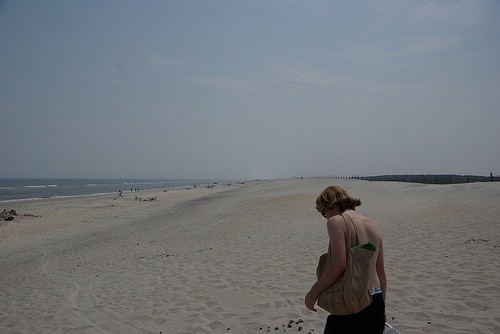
[319,204,331,218]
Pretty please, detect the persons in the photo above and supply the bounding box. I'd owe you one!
[305,186,388,334]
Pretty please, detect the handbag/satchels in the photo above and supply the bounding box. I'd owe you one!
[313,214,377,316]
